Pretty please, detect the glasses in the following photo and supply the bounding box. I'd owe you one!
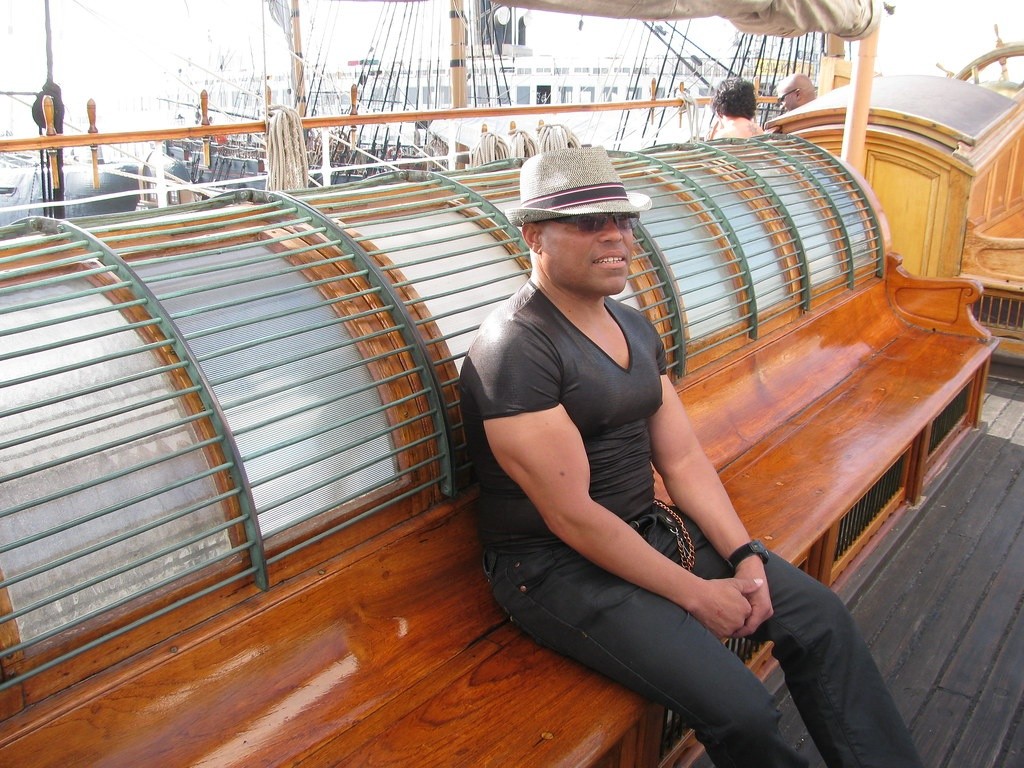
[778,88,799,103]
[545,212,641,233]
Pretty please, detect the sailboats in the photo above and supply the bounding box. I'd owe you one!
[0,0,830,230]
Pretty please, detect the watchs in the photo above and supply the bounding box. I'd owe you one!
[724,538,769,575]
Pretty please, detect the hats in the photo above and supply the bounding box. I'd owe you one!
[505,145,652,227]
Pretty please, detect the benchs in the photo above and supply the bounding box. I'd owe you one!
[1,131,1000,767]
[766,69,1024,365]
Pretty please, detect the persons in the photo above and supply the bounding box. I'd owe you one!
[778,74,816,111]
[710,78,764,139]
[460,146,924,768]
[209,116,214,125]
[215,135,231,168]
